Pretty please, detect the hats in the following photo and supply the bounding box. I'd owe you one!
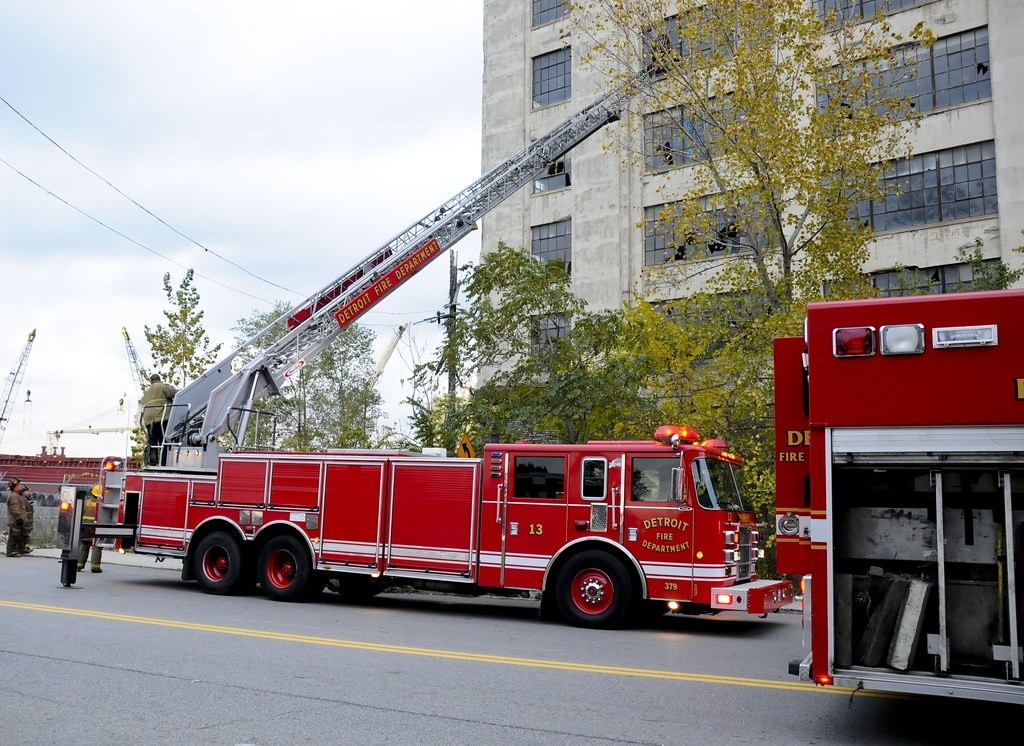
[19,486,29,492]
[8,477,21,488]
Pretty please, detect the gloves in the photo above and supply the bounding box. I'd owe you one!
[16,519,24,527]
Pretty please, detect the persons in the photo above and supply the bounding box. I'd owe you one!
[142,374,179,466]
[6,476,33,556]
[78,484,102,573]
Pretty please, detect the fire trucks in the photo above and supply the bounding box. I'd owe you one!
[770,289,1024,702]
[47,50,787,629]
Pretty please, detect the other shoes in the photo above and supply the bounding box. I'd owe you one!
[7,550,22,557]
[77,568,81,571]
[22,547,33,552]
[92,567,102,573]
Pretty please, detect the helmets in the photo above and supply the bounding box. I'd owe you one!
[90,485,102,497]
